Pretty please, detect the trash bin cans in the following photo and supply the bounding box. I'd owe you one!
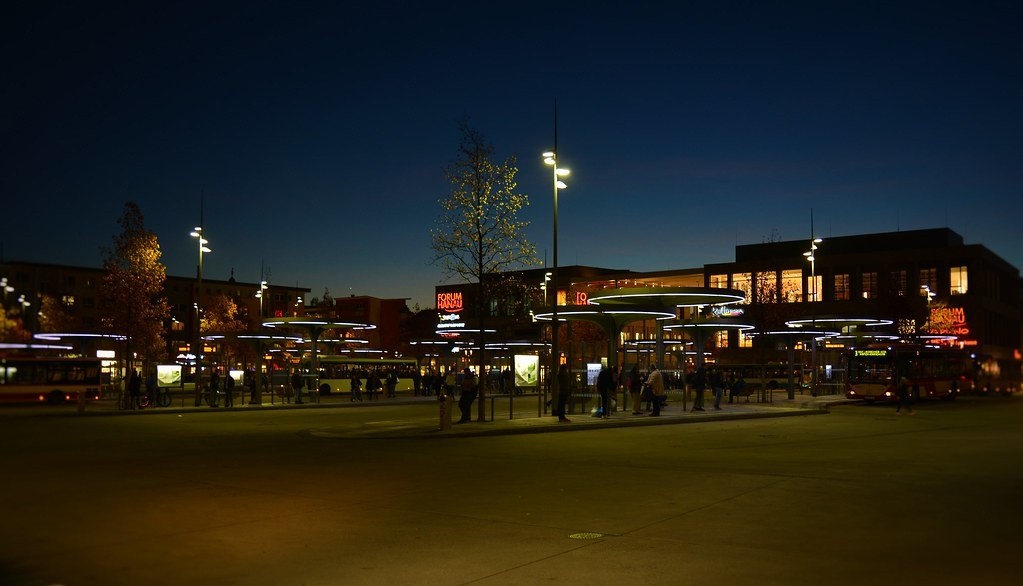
[439,395,451,430]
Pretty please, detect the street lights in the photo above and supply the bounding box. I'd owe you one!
[541,148,574,416]
[922,284,937,396]
[802,238,824,396]
[189,224,212,407]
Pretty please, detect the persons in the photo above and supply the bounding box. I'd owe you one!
[413,368,456,401]
[727,374,736,403]
[693,367,706,411]
[264,377,269,393]
[222,371,235,407]
[456,368,479,424]
[712,372,724,409]
[597,366,617,418]
[351,367,397,401]
[485,367,511,394]
[631,367,646,414]
[291,369,304,404]
[129,371,162,406]
[246,374,256,403]
[547,365,571,422]
[210,369,220,407]
[895,377,916,416]
[644,364,663,417]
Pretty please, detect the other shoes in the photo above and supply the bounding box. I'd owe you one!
[714,405,721,409]
[646,409,652,412]
[694,406,705,411]
[896,411,901,415]
[602,414,607,418]
[458,419,471,425]
[649,413,660,416]
[908,412,915,415]
[559,418,570,423]
[607,409,611,416]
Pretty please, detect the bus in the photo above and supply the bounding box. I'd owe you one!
[298,352,419,397]
[837,341,975,403]
[0,357,103,407]
[703,362,804,391]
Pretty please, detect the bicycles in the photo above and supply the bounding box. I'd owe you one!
[138,385,172,408]
